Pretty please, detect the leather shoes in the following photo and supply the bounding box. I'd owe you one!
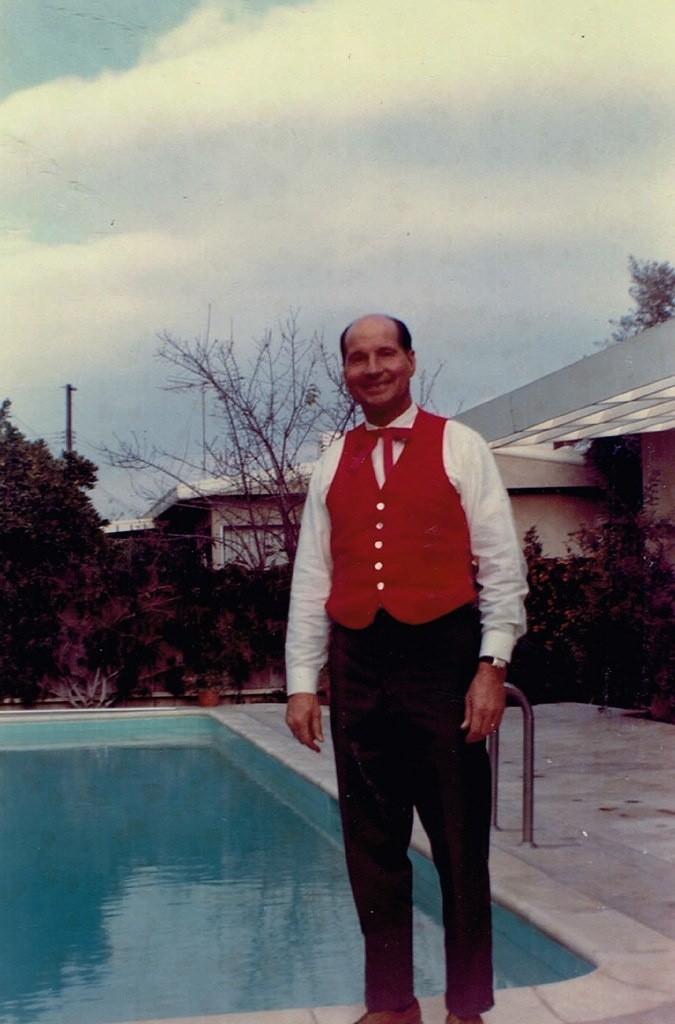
[355,998,423,1024]
[446,1013,484,1024]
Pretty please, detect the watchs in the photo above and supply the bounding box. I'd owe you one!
[478,656,509,672]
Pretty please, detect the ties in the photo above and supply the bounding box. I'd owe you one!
[351,427,412,495]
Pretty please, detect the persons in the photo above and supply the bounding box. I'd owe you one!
[284,313,530,1024]
